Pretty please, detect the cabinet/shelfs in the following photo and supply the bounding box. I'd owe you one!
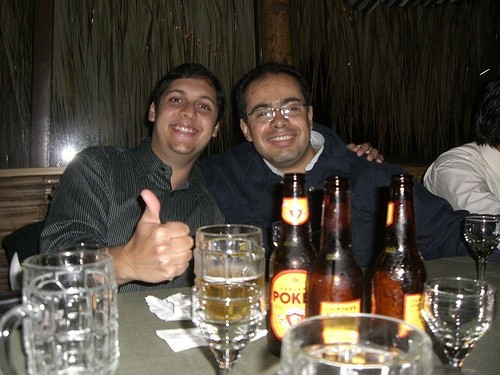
[0,249,500,375]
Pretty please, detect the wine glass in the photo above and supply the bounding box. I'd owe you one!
[463,216,500,294]
[422,276,495,375]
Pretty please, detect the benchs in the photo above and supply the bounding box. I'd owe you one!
[0,164,431,295]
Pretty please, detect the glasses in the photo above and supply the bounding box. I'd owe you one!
[246,103,311,122]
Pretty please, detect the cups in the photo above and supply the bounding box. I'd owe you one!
[190,223,264,375]
[280,312,435,375]
[0,251,120,375]
[272,222,312,248]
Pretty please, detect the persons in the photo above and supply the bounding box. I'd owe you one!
[420,78,500,251]
[206,62,500,275]
[38,61,385,294]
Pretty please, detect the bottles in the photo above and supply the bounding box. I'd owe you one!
[267,173,314,357]
[371,173,428,353]
[311,175,363,357]
